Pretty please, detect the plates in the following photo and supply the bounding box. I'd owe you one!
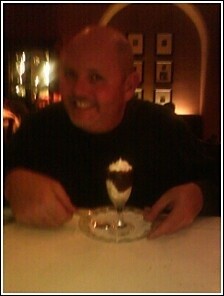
[89,206,160,240]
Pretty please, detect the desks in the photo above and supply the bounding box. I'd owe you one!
[3,205,222,294]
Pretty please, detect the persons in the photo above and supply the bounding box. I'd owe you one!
[3,25,221,240]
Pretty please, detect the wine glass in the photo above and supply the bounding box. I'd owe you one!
[105,166,133,233]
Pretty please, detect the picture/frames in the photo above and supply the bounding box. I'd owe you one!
[152,87,173,106]
[154,32,173,57]
[154,58,174,85]
[135,87,144,101]
[125,31,144,57]
[133,59,144,84]
